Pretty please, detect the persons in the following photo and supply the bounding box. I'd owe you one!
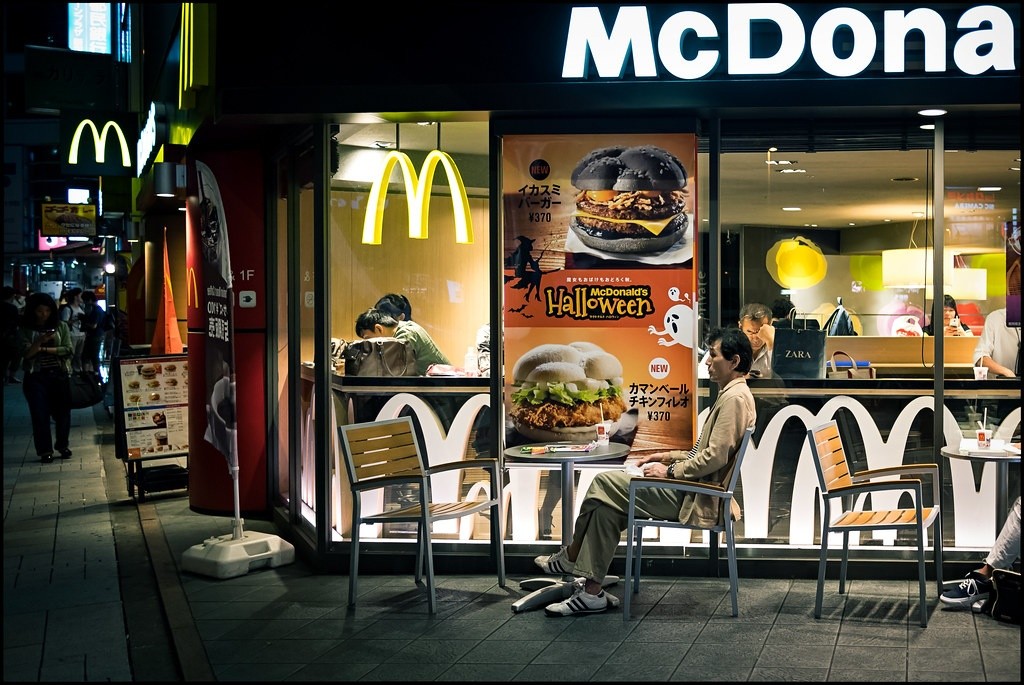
[940,496,1021,606]
[974,307,1021,411]
[3,286,102,462]
[541,328,756,617]
[922,295,973,336]
[353,293,577,540]
[699,303,774,378]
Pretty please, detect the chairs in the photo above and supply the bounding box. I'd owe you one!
[337,416,514,615]
[625,425,757,621]
[806,419,944,629]
[838,408,935,538]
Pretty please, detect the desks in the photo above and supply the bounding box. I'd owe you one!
[826,335,982,378]
[503,442,630,612]
[697,377,1021,397]
[300,361,493,454]
[940,444,1021,612]
[130,343,188,355]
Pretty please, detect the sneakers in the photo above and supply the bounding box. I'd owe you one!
[544,586,607,617]
[534,545,574,575]
[940,572,990,603]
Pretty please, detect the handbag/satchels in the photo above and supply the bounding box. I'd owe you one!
[826,350,876,380]
[68,346,106,408]
[893,315,923,337]
[346,338,408,377]
[771,305,826,379]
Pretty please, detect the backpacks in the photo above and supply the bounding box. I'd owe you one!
[822,296,858,336]
[99,312,116,332]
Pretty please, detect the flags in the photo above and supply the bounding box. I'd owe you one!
[196,159,233,463]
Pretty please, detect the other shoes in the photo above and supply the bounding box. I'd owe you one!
[54,444,72,457]
[40,454,53,463]
[8,377,23,386]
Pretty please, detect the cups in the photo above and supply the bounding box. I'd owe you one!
[596,423,611,446]
[976,429,992,448]
[964,405,998,431]
[154,431,167,446]
[972,367,988,379]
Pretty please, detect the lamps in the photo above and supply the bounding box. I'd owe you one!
[115,236,124,251]
[882,212,988,301]
[127,221,145,242]
[154,162,186,198]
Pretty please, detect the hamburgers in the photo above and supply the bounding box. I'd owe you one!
[508,342,627,441]
[129,363,189,402]
[568,145,688,254]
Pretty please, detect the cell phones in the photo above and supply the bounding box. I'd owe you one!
[950,319,960,329]
[46,329,55,336]
